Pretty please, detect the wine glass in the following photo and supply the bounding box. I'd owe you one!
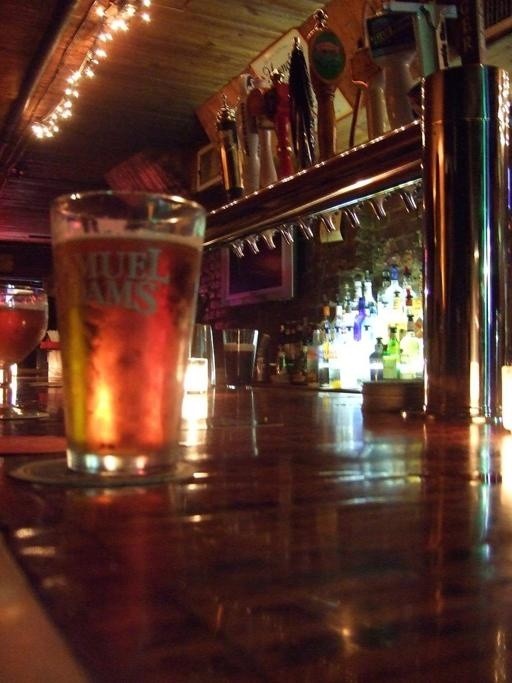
[0,281,50,417]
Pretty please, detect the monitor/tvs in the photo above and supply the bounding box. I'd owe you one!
[219,224,299,307]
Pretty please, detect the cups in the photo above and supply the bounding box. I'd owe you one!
[50,190,205,476]
[192,326,217,386]
[222,329,259,390]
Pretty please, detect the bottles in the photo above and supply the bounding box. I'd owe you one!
[272,263,424,390]
